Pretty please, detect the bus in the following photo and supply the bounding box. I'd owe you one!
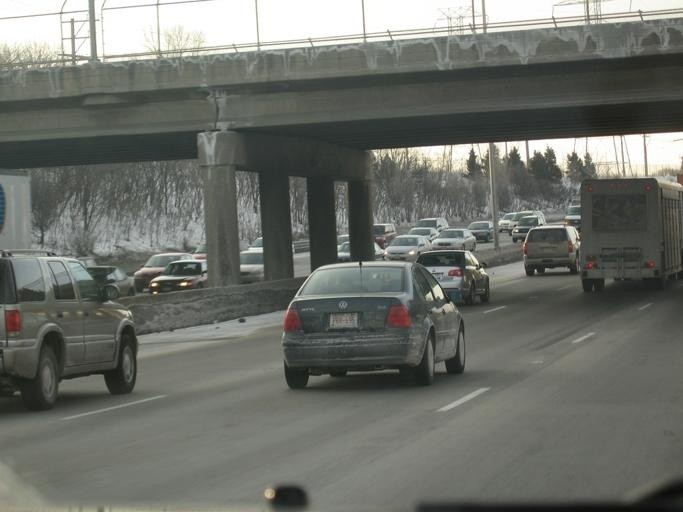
[577,175,682,295]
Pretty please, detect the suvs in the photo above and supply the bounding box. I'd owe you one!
[0,245,140,411]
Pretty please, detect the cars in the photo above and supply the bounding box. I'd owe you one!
[276,257,473,390]
